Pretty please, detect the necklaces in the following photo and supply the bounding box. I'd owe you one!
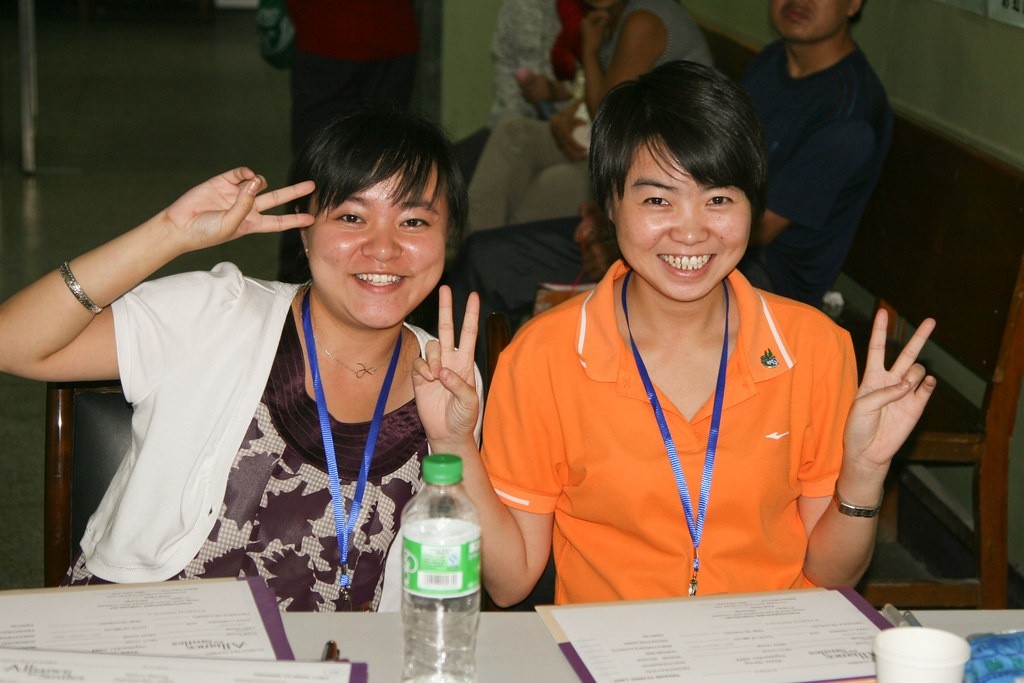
[300,306,397,379]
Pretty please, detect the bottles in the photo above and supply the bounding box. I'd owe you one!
[399,453,482,683]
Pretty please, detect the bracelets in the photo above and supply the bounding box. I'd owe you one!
[833,480,884,517]
[58,261,102,314]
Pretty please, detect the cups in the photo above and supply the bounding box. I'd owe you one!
[872,626,972,683]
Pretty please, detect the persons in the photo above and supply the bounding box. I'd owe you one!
[413,60,937,608]
[282,0,893,354]
[0,107,485,613]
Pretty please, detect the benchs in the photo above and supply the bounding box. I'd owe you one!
[513,13,1024,609]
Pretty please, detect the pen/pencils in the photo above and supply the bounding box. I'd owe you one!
[879,603,923,628]
[322,640,341,662]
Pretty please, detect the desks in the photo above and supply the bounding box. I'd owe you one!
[0,608,1024,683]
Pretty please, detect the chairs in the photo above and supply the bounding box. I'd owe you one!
[42,310,513,611]
